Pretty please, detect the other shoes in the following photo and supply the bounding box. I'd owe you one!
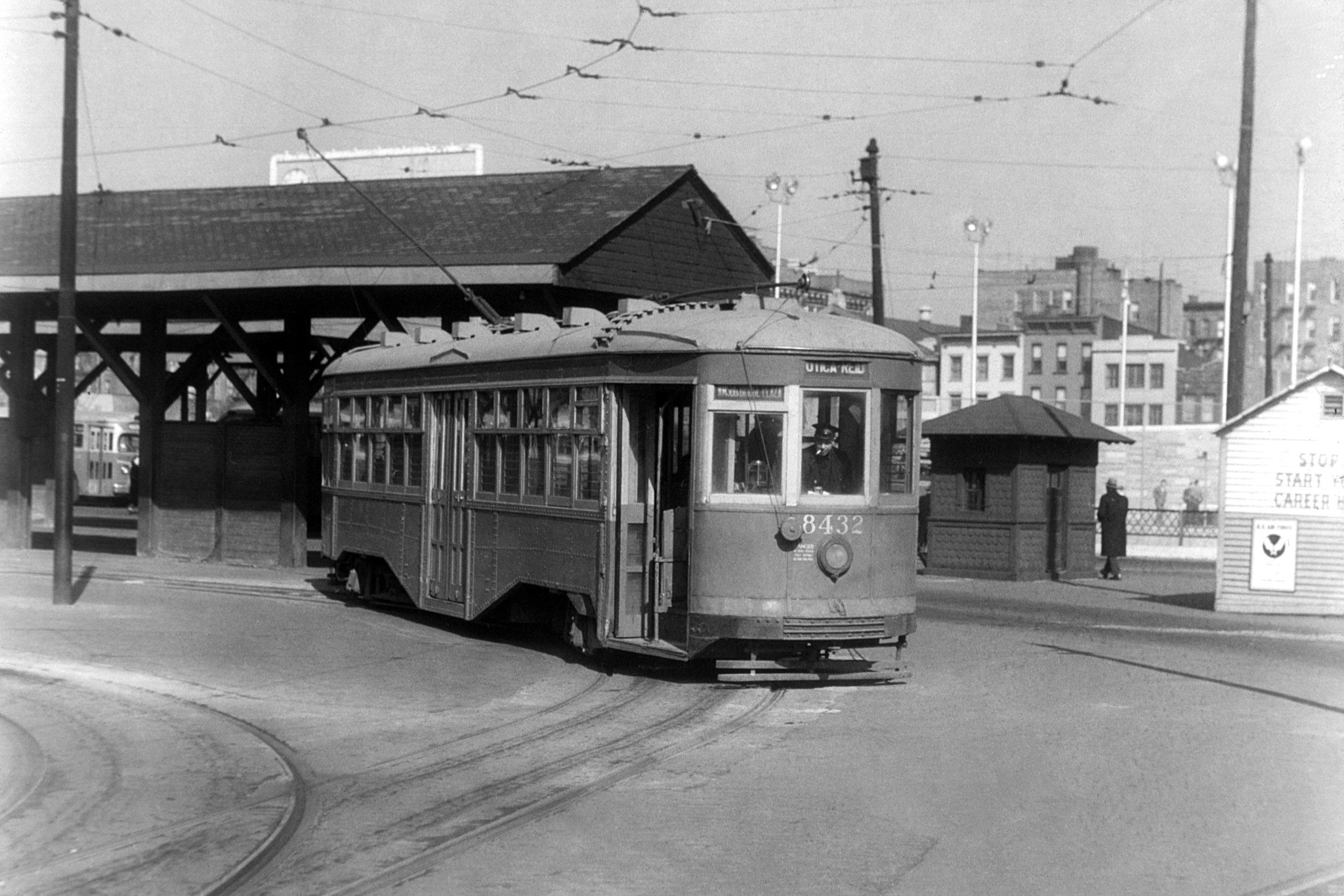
[1110,573,1120,580]
[1099,568,1107,578]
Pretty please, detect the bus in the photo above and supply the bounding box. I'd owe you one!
[295,127,923,685]
[72,421,140,505]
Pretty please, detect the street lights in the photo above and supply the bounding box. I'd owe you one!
[1289,137,1311,386]
[1213,153,1238,424]
[764,174,799,299]
[963,217,992,404]
[1118,266,1133,424]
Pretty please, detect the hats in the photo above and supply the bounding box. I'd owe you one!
[1103,477,1122,490]
[813,423,840,443]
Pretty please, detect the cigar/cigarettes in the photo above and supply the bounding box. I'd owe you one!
[816,450,821,454]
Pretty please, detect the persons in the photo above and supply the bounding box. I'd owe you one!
[128,456,140,513]
[738,413,782,481]
[1154,480,1167,519]
[1183,479,1202,518]
[1097,478,1129,579]
[802,422,854,495]
